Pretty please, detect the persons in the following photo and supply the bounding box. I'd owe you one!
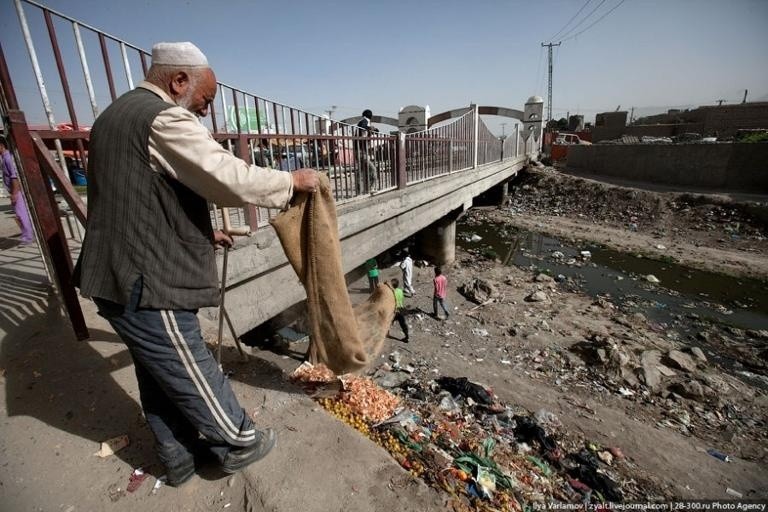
[429,266,451,319]
[388,279,409,344]
[352,109,379,194]
[0,136,36,246]
[68,40,323,487]
[399,251,416,296]
[363,257,379,293]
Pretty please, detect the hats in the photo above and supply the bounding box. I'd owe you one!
[152,41,209,67]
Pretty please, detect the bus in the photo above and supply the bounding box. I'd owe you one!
[733,128,768,141]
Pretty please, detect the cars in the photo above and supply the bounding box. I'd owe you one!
[556,138,570,145]
[280,143,313,166]
[638,131,719,145]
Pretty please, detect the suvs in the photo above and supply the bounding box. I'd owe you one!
[556,133,592,145]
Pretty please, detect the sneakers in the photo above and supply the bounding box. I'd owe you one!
[220,427,276,473]
[167,453,206,487]
